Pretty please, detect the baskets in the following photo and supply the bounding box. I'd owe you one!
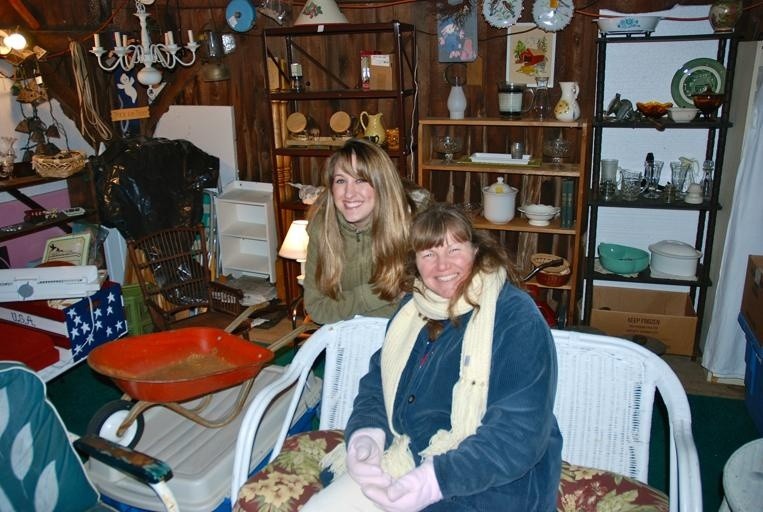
[31,151,85,178]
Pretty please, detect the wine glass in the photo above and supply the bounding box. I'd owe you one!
[432,135,464,167]
[542,140,571,171]
[692,93,726,122]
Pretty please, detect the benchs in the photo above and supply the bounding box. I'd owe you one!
[231,316,703,512]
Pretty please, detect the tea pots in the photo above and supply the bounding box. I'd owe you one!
[553,81,581,123]
[359,110,385,148]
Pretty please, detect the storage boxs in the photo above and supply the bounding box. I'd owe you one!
[741,254,763,346]
[738,312,763,437]
[82,364,323,512]
[591,286,698,358]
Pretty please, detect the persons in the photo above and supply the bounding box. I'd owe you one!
[343,200,564,512]
[302,136,416,325]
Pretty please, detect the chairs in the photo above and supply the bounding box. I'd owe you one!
[127,223,253,341]
[0,360,180,512]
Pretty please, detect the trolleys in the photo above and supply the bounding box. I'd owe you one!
[83,296,309,449]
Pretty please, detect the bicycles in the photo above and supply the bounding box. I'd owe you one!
[518,258,567,330]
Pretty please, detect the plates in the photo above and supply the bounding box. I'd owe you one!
[285,112,307,133]
[330,110,351,133]
[671,57,727,110]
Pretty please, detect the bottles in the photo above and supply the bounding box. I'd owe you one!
[480,177,519,227]
[707,0,744,35]
[684,159,715,206]
[445,85,468,119]
[599,154,699,208]
[531,76,552,121]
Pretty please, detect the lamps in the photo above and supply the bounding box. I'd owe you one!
[89,0,201,97]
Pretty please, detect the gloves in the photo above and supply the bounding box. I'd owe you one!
[346,427,444,512]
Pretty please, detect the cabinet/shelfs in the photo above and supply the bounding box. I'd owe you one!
[214,180,277,286]
[261,20,418,319]
[584,31,744,362]
[419,117,592,327]
[0,156,108,269]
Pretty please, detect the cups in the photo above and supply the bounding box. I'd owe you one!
[509,142,523,159]
[494,80,535,122]
[386,127,400,153]
[288,75,305,93]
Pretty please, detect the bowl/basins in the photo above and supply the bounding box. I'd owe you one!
[590,15,666,34]
[648,238,703,277]
[534,270,570,288]
[667,107,699,124]
[598,242,649,276]
[517,207,560,226]
[635,102,673,118]
